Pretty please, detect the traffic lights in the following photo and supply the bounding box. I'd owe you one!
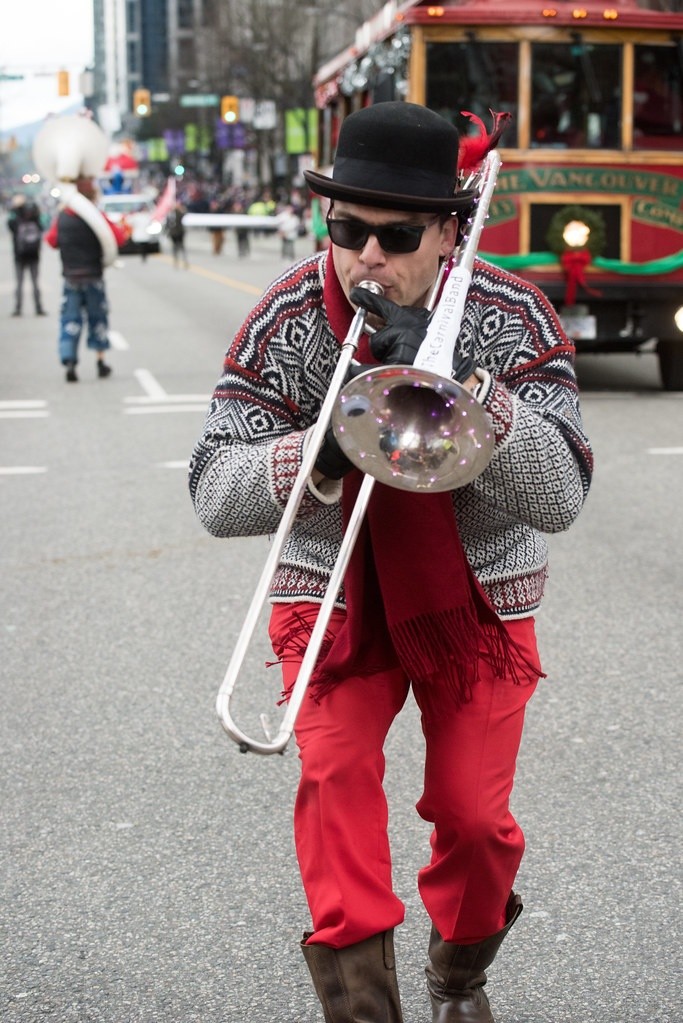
[132,88,152,118]
[220,95,241,126]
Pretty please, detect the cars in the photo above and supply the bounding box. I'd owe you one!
[95,190,162,255]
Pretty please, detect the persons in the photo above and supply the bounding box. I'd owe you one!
[43,183,135,380]
[188,101,594,1023]
[7,196,48,318]
[131,170,309,270]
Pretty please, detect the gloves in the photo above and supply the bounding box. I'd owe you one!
[349,287,479,385]
[312,365,376,481]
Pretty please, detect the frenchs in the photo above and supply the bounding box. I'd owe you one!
[30,114,120,266]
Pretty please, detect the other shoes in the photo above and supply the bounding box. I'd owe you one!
[66,369,78,382]
[99,367,111,375]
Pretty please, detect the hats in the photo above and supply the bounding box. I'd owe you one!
[303,101,479,213]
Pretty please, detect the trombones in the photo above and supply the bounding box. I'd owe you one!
[212,150,504,758]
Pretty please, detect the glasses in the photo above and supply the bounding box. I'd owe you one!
[325,204,441,254]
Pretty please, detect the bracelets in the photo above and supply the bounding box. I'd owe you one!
[469,382,482,400]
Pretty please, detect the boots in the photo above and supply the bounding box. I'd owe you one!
[300,932,403,1023]
[425,889,523,1023]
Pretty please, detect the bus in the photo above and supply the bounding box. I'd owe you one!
[307,0,683,394]
